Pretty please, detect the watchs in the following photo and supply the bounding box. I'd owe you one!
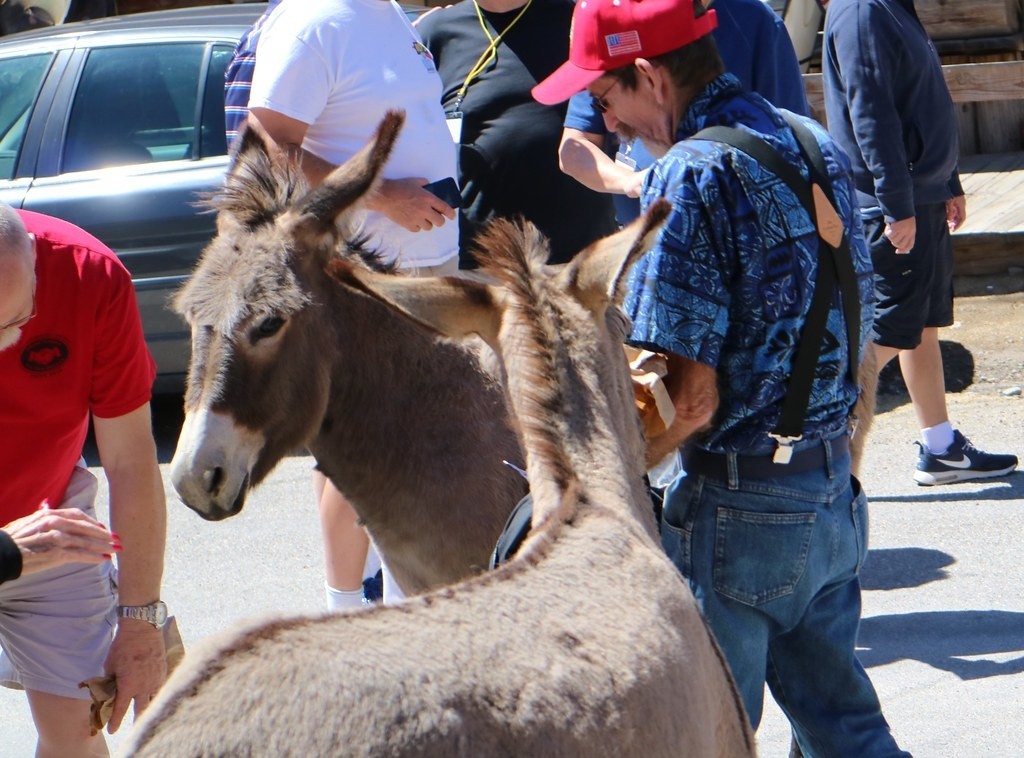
[116,601,167,629]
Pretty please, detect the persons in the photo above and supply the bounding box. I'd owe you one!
[0,200,169,758]
[532,0,910,758]
[562,0,810,230]
[821,0,1018,486]
[0,507,125,588]
[213,0,460,612]
[412,0,623,271]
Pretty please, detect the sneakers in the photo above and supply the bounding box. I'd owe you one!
[914,428,1020,485]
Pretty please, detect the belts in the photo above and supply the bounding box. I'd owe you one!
[681,428,851,480]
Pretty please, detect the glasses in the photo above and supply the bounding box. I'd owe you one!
[0,289,37,330]
[590,62,633,113]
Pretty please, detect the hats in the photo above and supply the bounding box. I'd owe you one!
[531,0,717,106]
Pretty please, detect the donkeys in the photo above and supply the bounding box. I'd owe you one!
[171,108,640,596]
[123,198,761,758]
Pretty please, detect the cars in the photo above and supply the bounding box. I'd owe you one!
[1,6,419,412]
